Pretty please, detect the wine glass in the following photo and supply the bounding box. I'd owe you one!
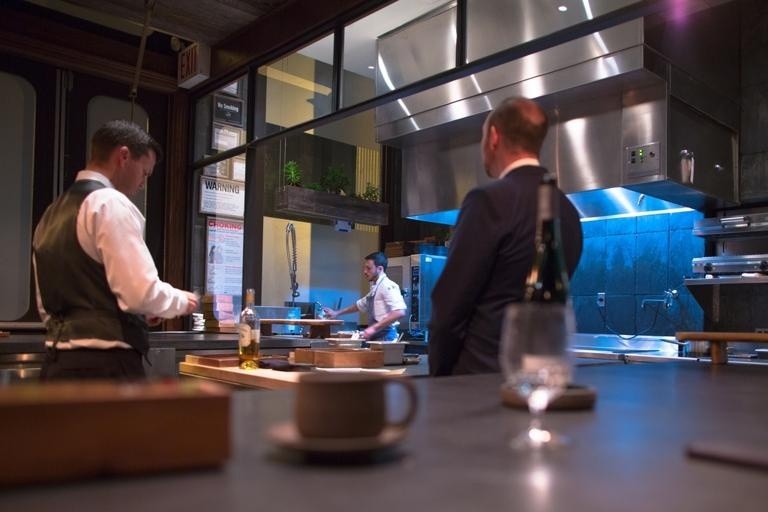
[499,298,578,448]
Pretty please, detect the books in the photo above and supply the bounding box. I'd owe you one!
[201,294,235,329]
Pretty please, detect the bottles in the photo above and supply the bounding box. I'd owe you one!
[237,288,260,370]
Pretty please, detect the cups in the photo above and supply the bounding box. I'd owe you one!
[297,371,418,434]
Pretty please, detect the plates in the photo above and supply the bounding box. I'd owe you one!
[325,338,365,348]
[269,420,401,461]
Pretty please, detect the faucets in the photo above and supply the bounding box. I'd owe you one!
[665,291,673,310]
[284,221,300,306]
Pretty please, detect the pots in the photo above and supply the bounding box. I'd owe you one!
[368,341,409,364]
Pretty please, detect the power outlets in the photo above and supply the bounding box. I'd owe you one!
[597,293,606,307]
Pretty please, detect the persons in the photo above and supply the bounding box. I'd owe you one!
[29,120,200,386]
[208,245,216,264]
[426,94,586,384]
[320,251,407,342]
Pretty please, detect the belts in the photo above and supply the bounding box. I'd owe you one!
[47,345,137,358]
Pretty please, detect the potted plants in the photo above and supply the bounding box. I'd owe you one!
[275,159,390,227]
